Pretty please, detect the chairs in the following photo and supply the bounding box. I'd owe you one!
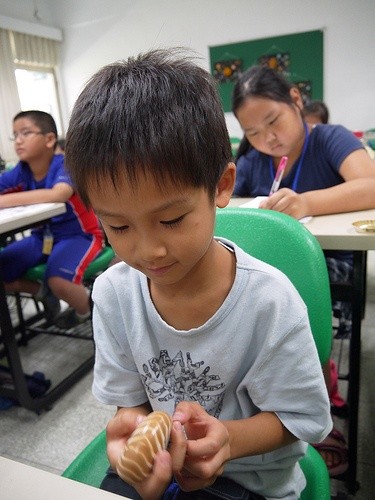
[61,207,333,500]
[15,247,115,346]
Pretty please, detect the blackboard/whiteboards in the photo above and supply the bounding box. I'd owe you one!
[208,29,324,113]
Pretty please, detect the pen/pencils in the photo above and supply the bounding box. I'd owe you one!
[270,156,288,195]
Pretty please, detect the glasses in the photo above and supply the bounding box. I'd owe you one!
[8,130,48,142]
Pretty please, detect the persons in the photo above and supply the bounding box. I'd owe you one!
[0,64,375,475]
[64,48,334,500]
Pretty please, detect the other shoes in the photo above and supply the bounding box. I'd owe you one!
[310,429,352,477]
[52,303,92,330]
[40,288,61,324]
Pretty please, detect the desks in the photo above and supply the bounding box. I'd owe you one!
[0,202,94,412]
[217,197,375,494]
